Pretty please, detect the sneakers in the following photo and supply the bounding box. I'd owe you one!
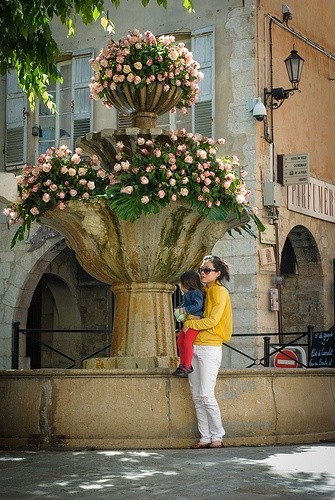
[178,366,193,377]
[172,364,184,375]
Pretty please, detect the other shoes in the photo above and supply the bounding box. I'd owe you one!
[209,442,223,448]
[190,443,211,449]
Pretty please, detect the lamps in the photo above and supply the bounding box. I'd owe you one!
[264,44,305,143]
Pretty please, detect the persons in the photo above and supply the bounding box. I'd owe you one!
[172,271,204,376]
[183,255,232,448]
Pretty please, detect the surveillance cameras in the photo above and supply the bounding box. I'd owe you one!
[253,97,268,121]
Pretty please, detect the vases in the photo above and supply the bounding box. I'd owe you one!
[36,196,250,369]
[76,128,203,172]
[105,83,184,128]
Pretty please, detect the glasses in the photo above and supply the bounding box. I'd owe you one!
[198,267,218,275]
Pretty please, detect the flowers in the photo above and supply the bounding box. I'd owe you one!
[88,29,204,114]
[3,126,266,250]
